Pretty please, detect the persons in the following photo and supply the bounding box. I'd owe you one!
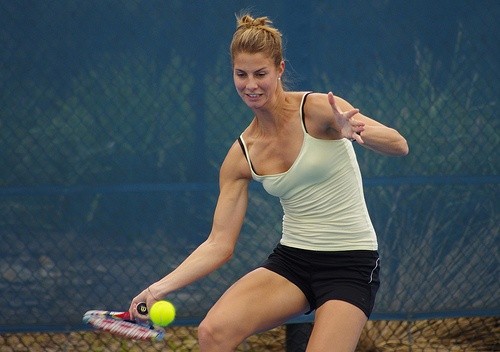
[129,12,408,352]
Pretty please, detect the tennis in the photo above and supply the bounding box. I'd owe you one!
[149,299,176,327]
[81,301,169,344]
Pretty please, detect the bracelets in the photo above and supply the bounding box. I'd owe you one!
[147,288,160,303]
[351,132,360,142]
